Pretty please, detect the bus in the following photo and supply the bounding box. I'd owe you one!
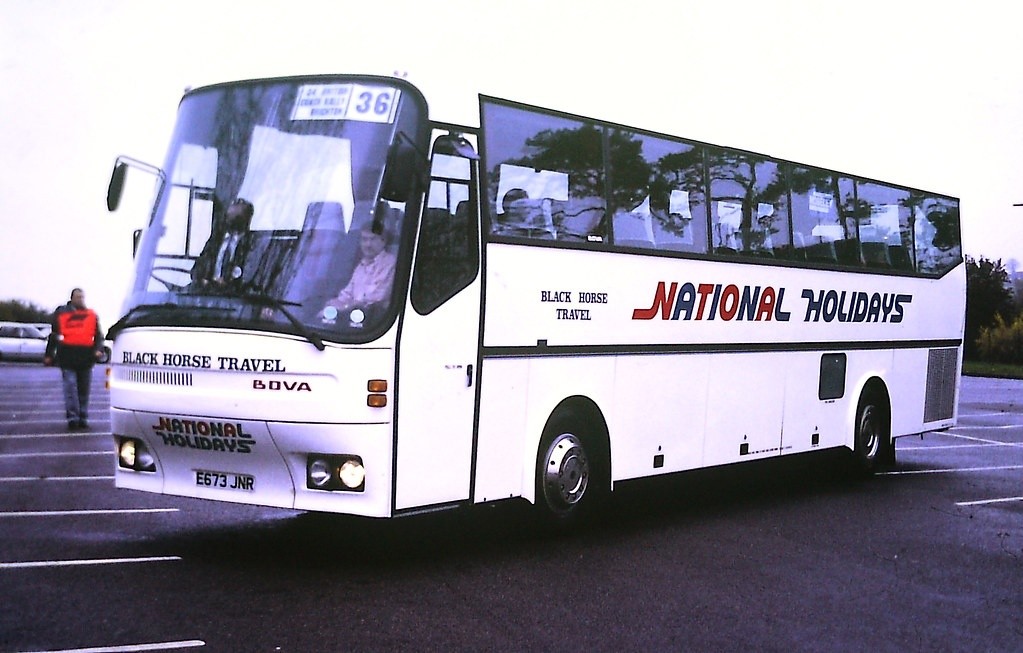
[102,70,970,533]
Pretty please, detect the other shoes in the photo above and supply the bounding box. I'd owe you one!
[69,422,91,434]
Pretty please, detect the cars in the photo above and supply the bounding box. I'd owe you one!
[0,321,57,360]
[39,324,113,364]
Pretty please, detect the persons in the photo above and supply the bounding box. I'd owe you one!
[44,289,103,430]
[188,200,265,294]
[314,219,399,315]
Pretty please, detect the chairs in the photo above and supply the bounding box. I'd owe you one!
[272,199,915,292]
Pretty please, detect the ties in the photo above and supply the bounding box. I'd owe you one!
[221,234,232,279]
